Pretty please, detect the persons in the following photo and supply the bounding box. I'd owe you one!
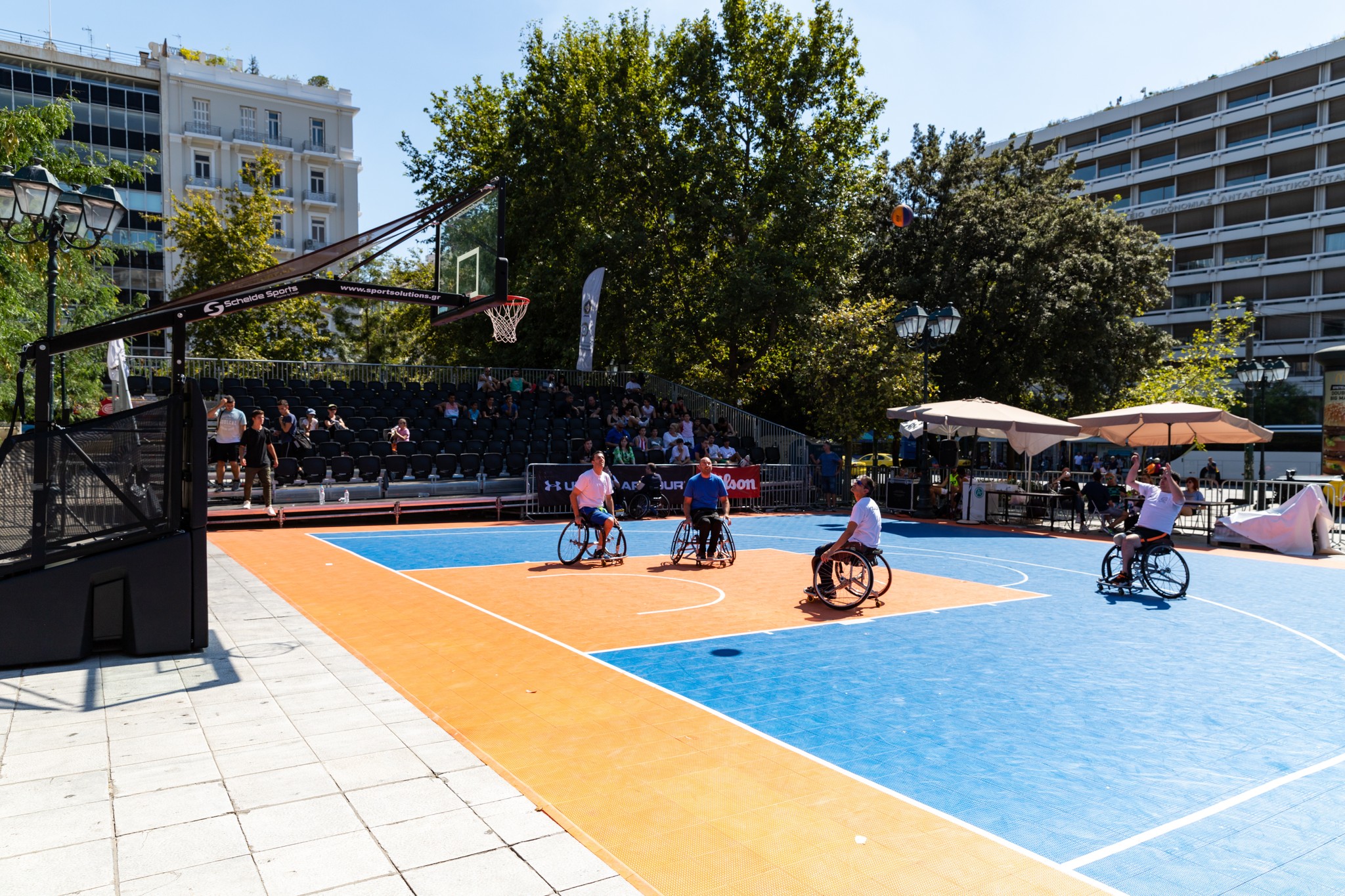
[1108,452,1186,584]
[804,476,882,599]
[208,367,752,514]
[570,451,619,558]
[683,457,732,560]
[895,457,1217,522]
[810,441,843,511]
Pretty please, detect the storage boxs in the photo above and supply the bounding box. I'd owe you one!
[886,478,920,517]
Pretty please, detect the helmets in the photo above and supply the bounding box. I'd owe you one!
[1146,456,1160,464]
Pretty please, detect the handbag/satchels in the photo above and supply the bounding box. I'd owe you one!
[292,433,312,450]
[1096,467,1106,474]
[1010,487,1029,505]
[208,438,217,464]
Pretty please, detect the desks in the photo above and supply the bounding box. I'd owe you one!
[984,490,1074,533]
[1123,498,1233,544]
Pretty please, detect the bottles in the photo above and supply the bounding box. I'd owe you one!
[319,485,324,495]
[391,425,399,433]
[338,497,345,501]
[528,482,530,494]
[1058,484,1061,492]
[319,490,325,505]
[344,490,349,503]
[418,492,431,497]
[905,470,908,478]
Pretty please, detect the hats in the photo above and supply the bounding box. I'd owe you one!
[306,408,316,415]
[328,404,337,407]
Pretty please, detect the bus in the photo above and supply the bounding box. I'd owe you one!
[1164,424,1322,486]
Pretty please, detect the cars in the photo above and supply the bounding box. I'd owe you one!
[858,453,904,474]
[842,455,866,479]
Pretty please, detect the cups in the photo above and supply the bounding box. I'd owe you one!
[1066,472,1070,477]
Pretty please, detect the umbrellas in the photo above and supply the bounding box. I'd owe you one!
[886,397,1101,520]
[1067,399,1274,468]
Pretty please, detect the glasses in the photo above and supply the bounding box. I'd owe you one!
[615,408,618,410]
[1106,476,1113,481]
[1186,481,1192,484]
[857,480,866,489]
[629,411,632,412]
[329,408,336,411]
[899,469,904,472]
[486,370,490,372]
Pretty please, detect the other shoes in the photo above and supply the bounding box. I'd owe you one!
[832,507,836,512]
[264,504,276,516]
[1208,486,1212,490]
[231,479,241,491]
[814,583,837,599]
[214,484,225,493]
[824,507,831,511]
[707,553,715,557]
[739,455,749,467]
[242,500,252,509]
[1079,525,1090,532]
[1076,516,1081,523]
[593,548,611,558]
[1103,526,1117,536]
[698,551,706,559]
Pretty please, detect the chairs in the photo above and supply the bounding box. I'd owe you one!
[125,375,781,488]
[1001,496,1112,535]
[1178,508,1205,536]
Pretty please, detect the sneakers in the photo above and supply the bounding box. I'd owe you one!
[1111,570,1132,586]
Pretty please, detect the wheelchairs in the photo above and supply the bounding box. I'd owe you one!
[629,484,670,520]
[1096,538,1190,600]
[603,487,630,521]
[806,542,892,610]
[670,512,736,568]
[557,507,628,568]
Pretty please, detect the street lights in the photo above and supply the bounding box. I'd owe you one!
[1235,355,1292,503]
[1,156,130,535]
[891,299,963,517]
